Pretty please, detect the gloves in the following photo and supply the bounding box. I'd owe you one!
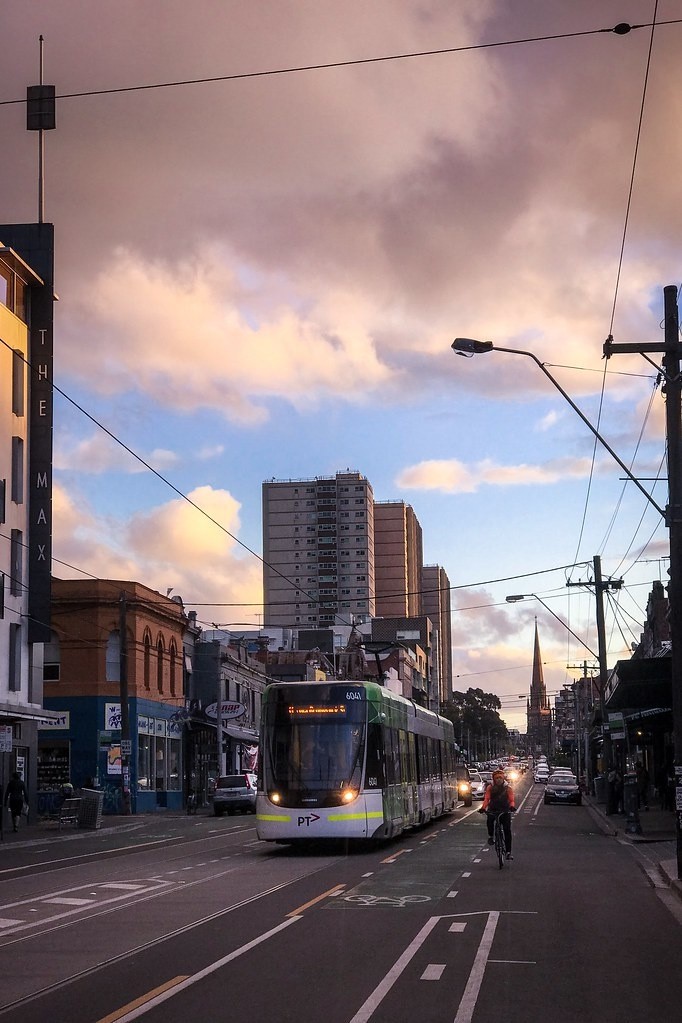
[510,806,514,811]
[479,809,485,814]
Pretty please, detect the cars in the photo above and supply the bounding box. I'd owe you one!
[532,755,573,784]
[468,757,529,801]
[457,765,474,807]
[213,774,258,817]
[544,774,583,806]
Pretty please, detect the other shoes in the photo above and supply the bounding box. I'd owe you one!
[506,854,513,860]
[488,837,494,846]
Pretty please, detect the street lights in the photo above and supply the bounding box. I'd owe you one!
[452,284,682,881]
[506,556,609,723]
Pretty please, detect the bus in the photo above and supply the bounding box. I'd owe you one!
[256,680,458,847]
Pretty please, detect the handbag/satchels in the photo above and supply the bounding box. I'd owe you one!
[22,802,29,816]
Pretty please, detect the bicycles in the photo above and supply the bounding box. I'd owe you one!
[478,808,514,870]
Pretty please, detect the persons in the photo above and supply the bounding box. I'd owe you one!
[59,778,73,803]
[479,770,516,860]
[4,772,29,832]
[635,761,651,811]
